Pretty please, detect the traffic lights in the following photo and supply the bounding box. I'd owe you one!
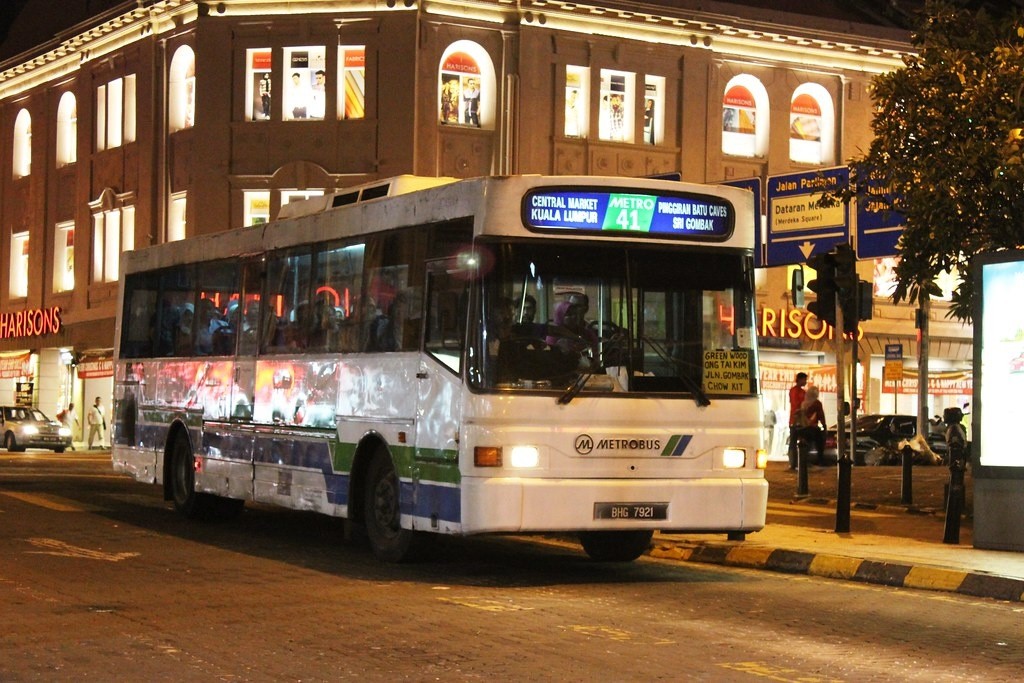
[823,242,856,311]
[802,250,835,327]
[858,281,874,321]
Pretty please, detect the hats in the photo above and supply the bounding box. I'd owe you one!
[69,403,74,407]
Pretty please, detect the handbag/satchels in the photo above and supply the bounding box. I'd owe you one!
[56,410,67,423]
[792,402,809,429]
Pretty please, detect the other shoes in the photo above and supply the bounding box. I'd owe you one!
[100,446,106,450]
[89,447,92,450]
[72,447,75,451]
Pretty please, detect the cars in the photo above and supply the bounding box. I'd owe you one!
[0,403,73,455]
[788,413,951,472]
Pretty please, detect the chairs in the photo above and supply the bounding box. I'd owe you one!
[163,307,632,379]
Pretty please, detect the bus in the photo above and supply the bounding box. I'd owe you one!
[108,170,806,567]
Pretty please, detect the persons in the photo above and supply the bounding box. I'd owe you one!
[57,403,80,451]
[147,290,607,383]
[762,409,776,454]
[438,78,480,128]
[787,372,969,518]
[306,70,325,117]
[258,72,271,118]
[87,396,108,450]
[609,94,653,142]
[288,74,308,119]
